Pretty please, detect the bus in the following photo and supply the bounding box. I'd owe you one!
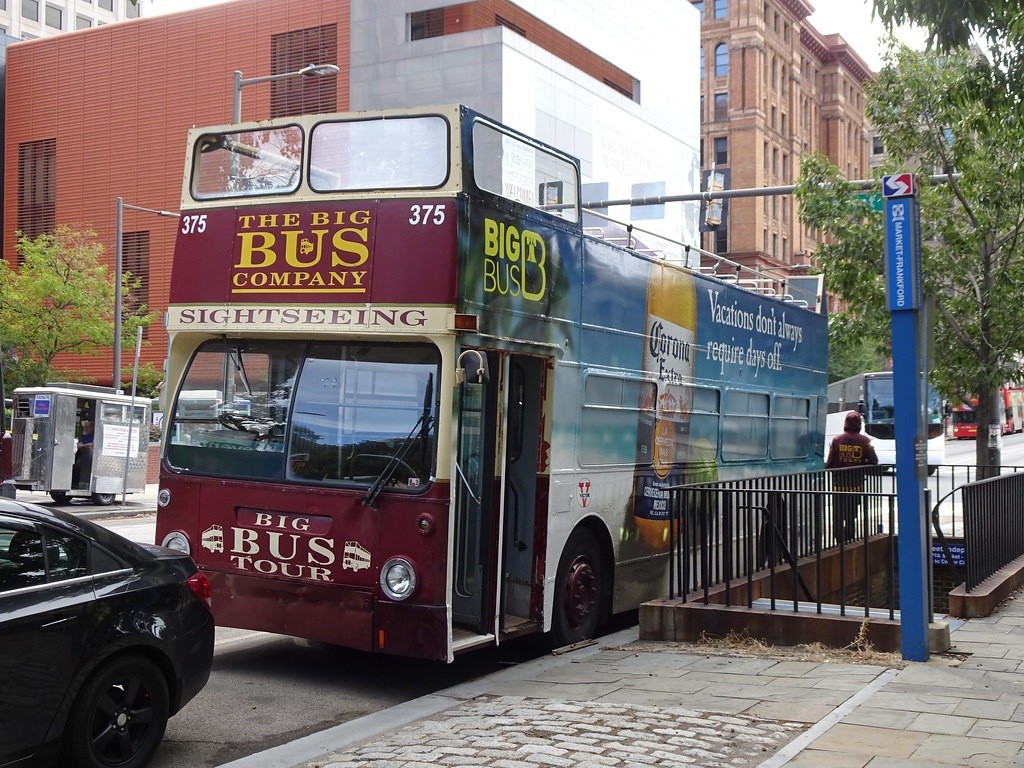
[944,387,1024,439]
[823,369,947,475]
[153,105,839,666]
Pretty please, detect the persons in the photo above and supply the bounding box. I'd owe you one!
[825,412,878,542]
[76,411,95,481]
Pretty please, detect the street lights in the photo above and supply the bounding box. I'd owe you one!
[229,61,340,194]
[112,195,181,389]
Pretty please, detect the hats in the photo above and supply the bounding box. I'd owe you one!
[79,410,89,420]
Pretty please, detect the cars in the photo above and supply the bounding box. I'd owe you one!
[1,498,217,768]
